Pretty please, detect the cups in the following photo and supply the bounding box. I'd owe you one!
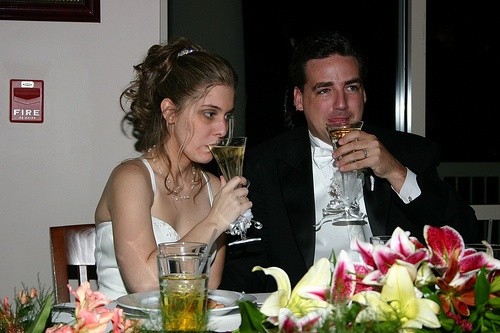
[153,240,207,333]
[369,236,390,244]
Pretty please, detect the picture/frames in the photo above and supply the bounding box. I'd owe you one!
[0,0,100,23]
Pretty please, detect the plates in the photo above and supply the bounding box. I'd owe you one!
[116,290,257,316]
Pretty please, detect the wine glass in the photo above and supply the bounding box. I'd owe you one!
[325,121,365,223]
[208,136,262,246]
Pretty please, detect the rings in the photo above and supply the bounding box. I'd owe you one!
[362,148,367,159]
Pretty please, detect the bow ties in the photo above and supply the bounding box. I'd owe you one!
[315,146,334,170]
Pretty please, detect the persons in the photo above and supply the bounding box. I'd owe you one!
[234,32,478,294]
[90,37,252,303]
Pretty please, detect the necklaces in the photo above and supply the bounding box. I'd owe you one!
[145,149,196,201]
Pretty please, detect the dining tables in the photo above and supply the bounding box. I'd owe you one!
[51,293,272,333]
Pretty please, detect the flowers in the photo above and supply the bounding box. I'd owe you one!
[0,271,221,333]
[236,225,500,333]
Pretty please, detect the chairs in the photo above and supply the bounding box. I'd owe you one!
[50,224,97,304]
